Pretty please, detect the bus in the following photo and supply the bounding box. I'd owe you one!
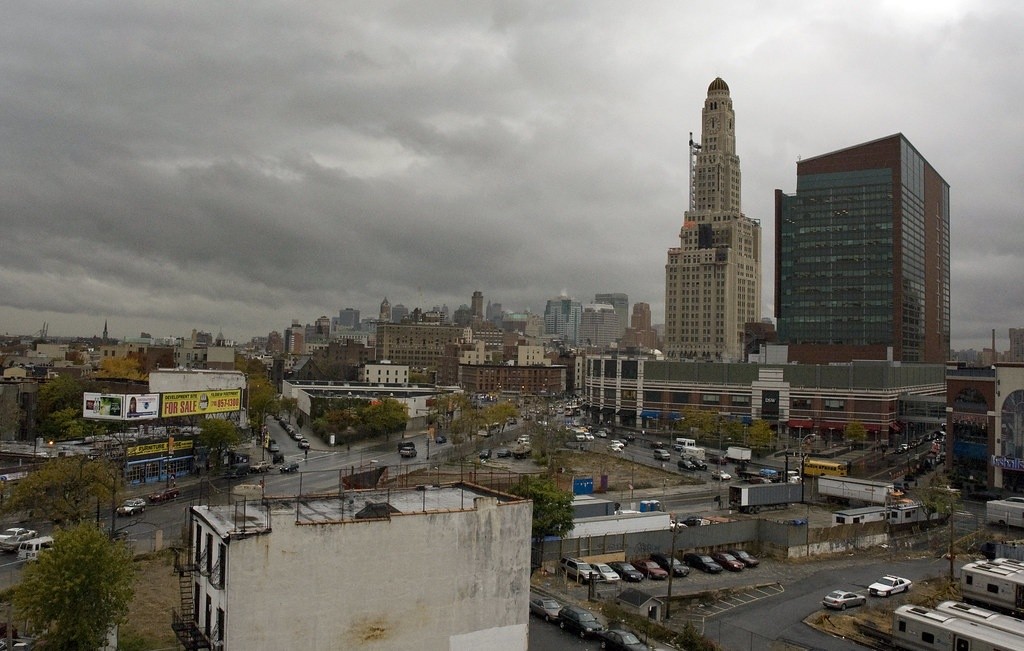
[804,460,847,478]
[477,422,501,437]
[564,406,580,416]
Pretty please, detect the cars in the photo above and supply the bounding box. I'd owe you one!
[930,484,963,496]
[712,471,731,481]
[479,449,492,459]
[894,430,940,454]
[1005,497,1024,503]
[728,549,760,567]
[632,559,668,580]
[269,441,279,453]
[867,575,912,597]
[680,517,702,526]
[610,440,624,449]
[594,630,650,651]
[690,459,708,470]
[670,520,687,529]
[279,461,299,473]
[400,447,417,458]
[606,445,622,453]
[48,507,89,524]
[250,461,272,474]
[624,433,636,440]
[437,437,447,443]
[609,561,644,582]
[518,435,531,444]
[678,460,696,470]
[116,498,146,516]
[684,553,723,574]
[651,441,663,448]
[0,528,39,542]
[279,419,303,441]
[710,551,744,572]
[558,605,605,639]
[655,555,690,576]
[148,487,180,503]
[273,453,284,464]
[590,562,621,583]
[298,439,310,449]
[497,449,511,458]
[529,597,563,622]
[523,415,532,421]
[570,420,595,441]
[0,535,35,552]
[398,442,415,453]
[823,590,866,610]
[508,418,517,424]
[595,431,607,438]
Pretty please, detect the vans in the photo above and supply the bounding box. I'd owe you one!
[15,536,54,562]
[654,449,670,461]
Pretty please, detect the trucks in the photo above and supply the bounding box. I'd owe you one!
[673,438,695,451]
[680,447,706,461]
[986,500,1024,528]
[740,469,801,485]
[223,463,250,480]
[724,447,752,464]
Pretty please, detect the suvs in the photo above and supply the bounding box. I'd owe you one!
[560,556,600,584]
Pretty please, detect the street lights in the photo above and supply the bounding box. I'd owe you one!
[670,417,684,448]
[906,451,910,474]
[829,427,836,449]
[166,454,173,487]
[347,395,352,451]
[719,438,731,481]
[799,428,815,477]
[666,515,682,619]
[262,399,279,461]
[369,460,378,488]
[875,430,878,452]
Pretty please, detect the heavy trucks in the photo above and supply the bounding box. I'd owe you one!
[818,475,915,507]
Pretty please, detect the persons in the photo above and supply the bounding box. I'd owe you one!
[127,397,139,418]
